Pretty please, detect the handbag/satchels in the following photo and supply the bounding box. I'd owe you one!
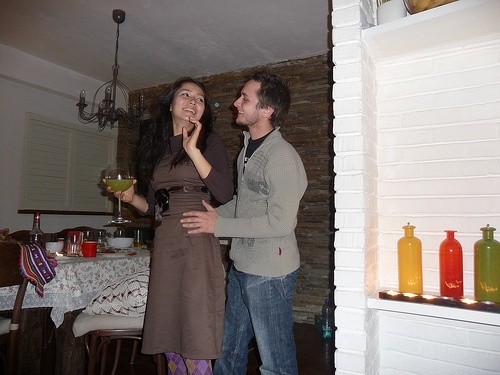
[0,239,29,288]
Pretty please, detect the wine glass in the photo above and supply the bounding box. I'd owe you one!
[105,161,134,223]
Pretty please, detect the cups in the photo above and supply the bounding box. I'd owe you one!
[67,231,81,256]
[82,241,97,258]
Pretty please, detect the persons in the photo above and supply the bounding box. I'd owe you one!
[103,76,235,375]
[181,72,309,375]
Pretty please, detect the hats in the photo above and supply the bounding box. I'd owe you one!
[17,242,56,296]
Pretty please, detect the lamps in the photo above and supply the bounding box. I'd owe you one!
[76,9,147,133]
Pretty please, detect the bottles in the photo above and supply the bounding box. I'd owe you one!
[29,212,44,248]
[473,226,500,302]
[322,298,334,338]
[439,230,463,299]
[397,226,422,294]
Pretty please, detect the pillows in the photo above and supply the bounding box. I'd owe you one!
[82,266,150,317]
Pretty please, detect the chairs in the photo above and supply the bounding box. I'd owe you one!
[73,265,168,374]
[0,240,56,375]
[0,225,107,242]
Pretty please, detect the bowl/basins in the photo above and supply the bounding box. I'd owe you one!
[46,242,64,253]
[105,237,135,249]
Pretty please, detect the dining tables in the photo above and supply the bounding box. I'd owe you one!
[0,239,156,375]
